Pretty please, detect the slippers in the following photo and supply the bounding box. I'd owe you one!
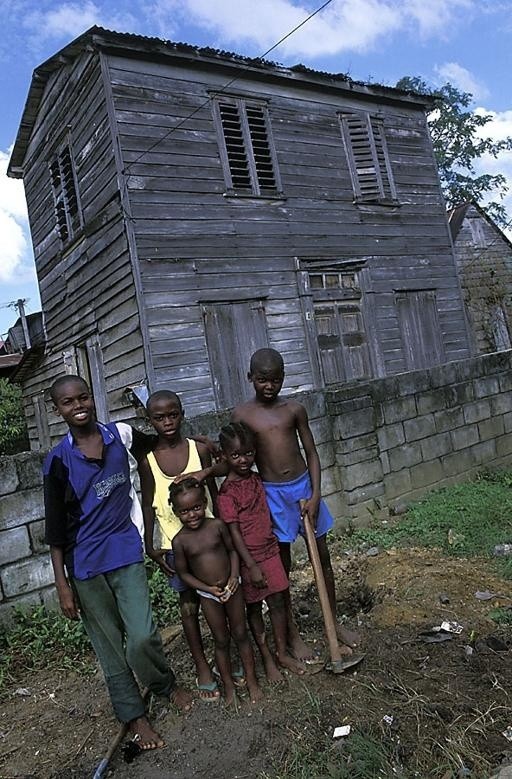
[193,673,221,703]
[233,654,249,689]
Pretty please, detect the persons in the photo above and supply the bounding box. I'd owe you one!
[167,479,264,709]
[44,375,224,750]
[136,389,246,702]
[217,421,307,685]
[173,347,360,664]
